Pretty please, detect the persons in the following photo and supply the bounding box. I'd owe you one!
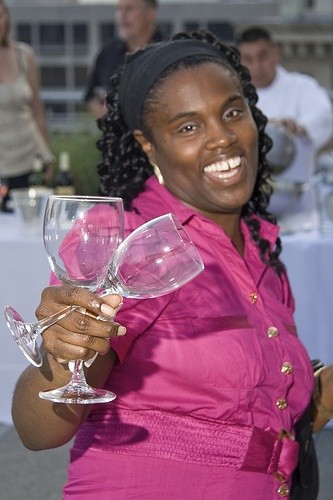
[11,30,333,500]
[240,25,333,212]
[0,0,53,189]
[85,0,169,121]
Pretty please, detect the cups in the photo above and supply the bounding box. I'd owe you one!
[7,186,53,236]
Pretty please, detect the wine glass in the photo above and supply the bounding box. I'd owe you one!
[39,194,125,406]
[2,211,206,367]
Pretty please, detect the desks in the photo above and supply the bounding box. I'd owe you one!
[0,213,333,428]
